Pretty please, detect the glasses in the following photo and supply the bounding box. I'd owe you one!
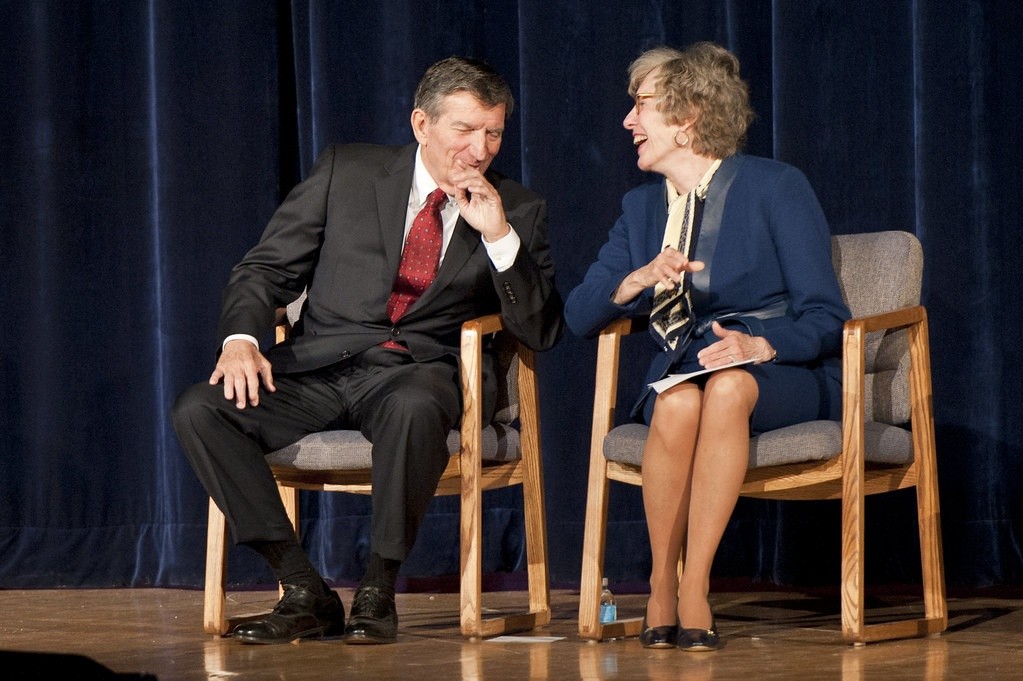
[634,92,664,115]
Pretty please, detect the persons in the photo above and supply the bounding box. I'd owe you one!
[559,43,854,655]
[170,56,565,647]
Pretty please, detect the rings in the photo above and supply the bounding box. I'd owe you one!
[728,353,737,363]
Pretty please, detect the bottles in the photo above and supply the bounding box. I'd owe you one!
[600,578,616,625]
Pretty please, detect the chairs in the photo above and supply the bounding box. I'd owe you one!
[577,232,943,642]
[200,288,552,635]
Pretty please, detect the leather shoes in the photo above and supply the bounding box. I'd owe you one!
[676,605,719,652]
[639,604,677,649]
[233,583,346,644]
[342,585,399,644]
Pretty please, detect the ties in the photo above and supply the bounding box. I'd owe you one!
[378,188,450,350]
